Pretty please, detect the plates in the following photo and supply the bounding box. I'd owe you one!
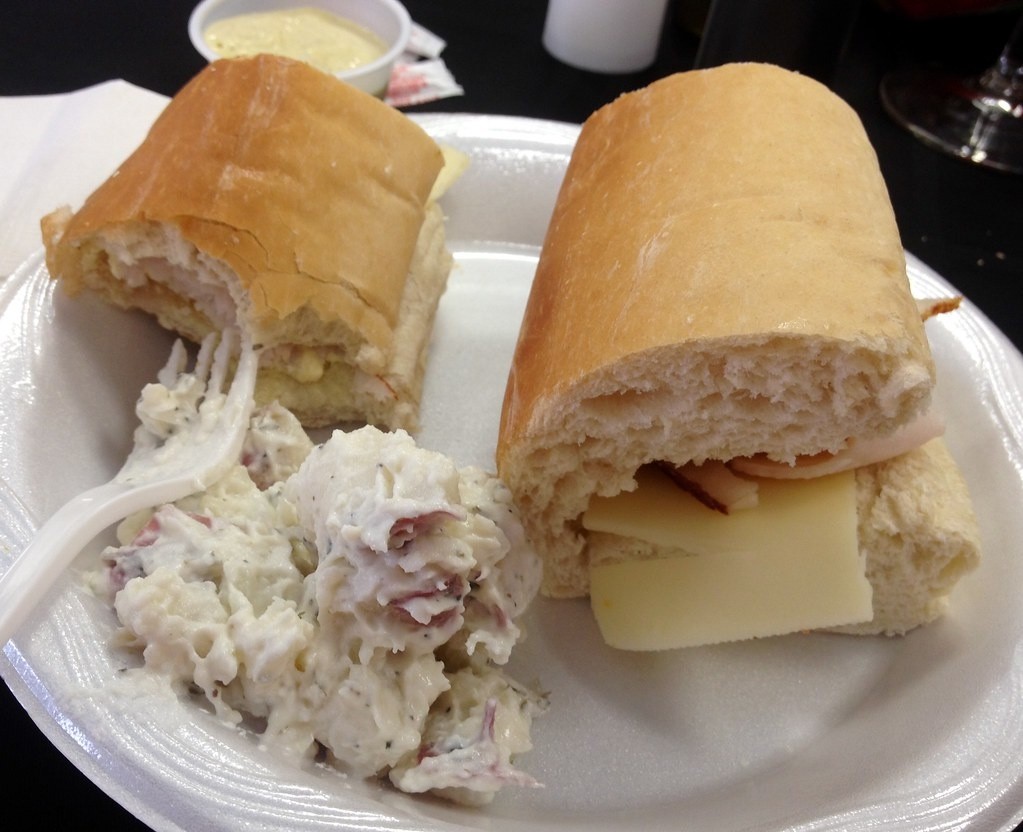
[0,115,1023,832]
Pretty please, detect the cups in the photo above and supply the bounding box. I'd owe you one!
[542,0,666,73]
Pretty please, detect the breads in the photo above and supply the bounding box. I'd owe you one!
[489,61,980,653]
[38,55,470,437]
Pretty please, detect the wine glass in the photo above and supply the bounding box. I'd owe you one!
[880,0,1023,173]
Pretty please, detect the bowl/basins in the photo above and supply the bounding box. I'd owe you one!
[188,0,412,99]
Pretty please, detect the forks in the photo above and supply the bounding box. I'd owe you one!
[0,327,260,653]
